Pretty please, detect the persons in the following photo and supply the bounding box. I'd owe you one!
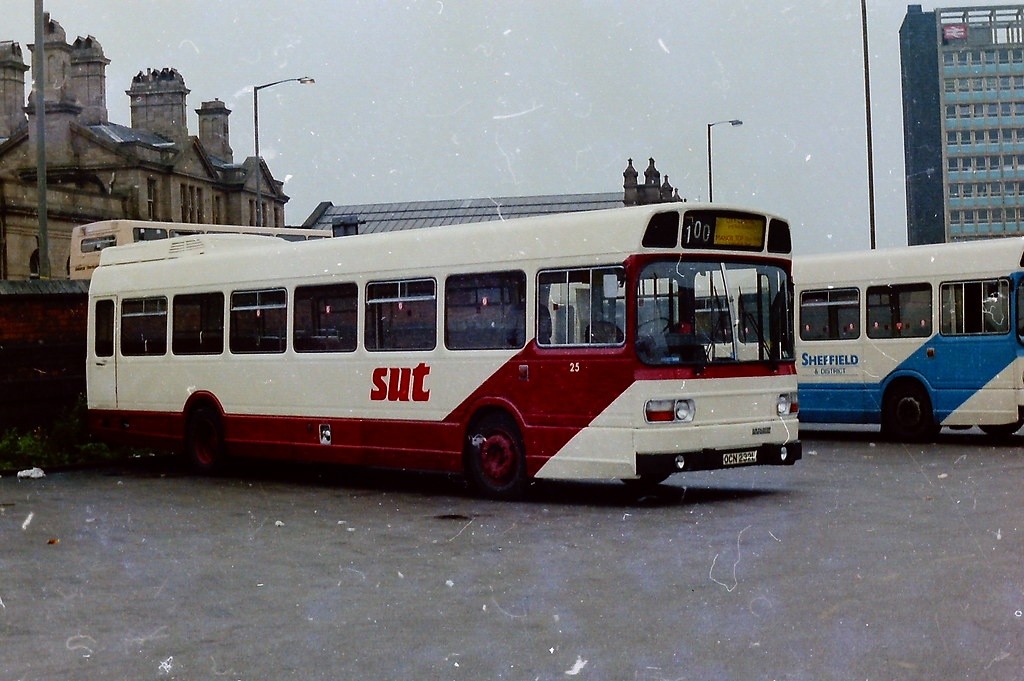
[505,290,552,347]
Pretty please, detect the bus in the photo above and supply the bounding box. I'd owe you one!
[69,219,332,279]
[550,236,1024,442]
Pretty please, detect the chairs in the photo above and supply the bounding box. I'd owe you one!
[585,320,623,345]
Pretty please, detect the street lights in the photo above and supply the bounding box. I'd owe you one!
[708,120,743,202]
[85,201,802,496]
[254,76,315,226]
